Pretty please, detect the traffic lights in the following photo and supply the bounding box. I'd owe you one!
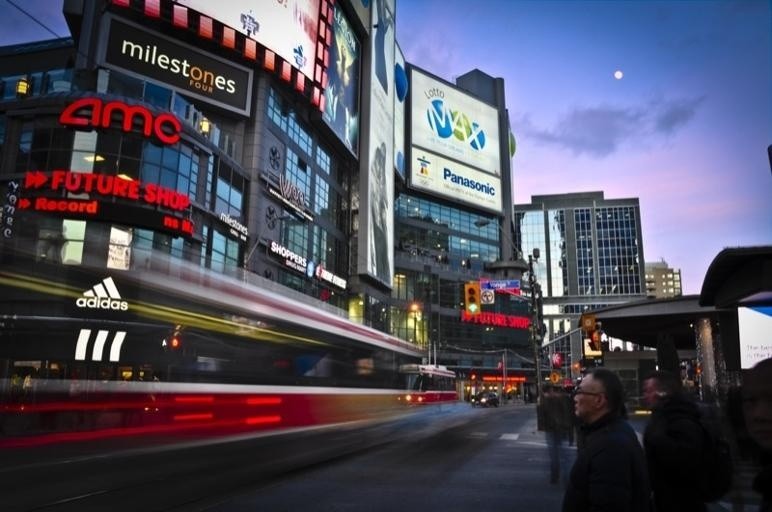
[469,371,477,381]
[465,283,481,314]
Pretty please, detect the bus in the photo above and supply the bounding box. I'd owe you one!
[403,364,459,414]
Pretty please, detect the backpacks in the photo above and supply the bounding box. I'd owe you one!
[697,438,735,505]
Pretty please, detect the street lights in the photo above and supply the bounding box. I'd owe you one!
[474,219,544,398]
[412,304,418,344]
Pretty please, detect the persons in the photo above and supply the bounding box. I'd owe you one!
[537,358,772,511]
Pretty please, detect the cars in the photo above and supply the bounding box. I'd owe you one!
[471,392,500,408]
